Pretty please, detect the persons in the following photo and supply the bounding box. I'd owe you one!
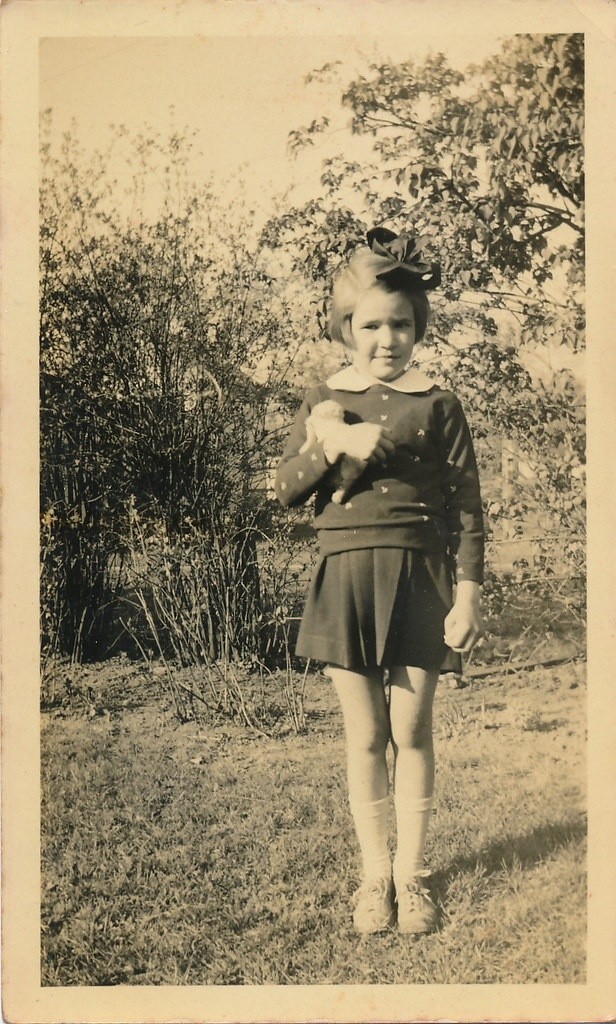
[275,228,483,934]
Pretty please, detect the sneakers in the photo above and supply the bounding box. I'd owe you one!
[351,872,394,932]
[396,873,439,932]
